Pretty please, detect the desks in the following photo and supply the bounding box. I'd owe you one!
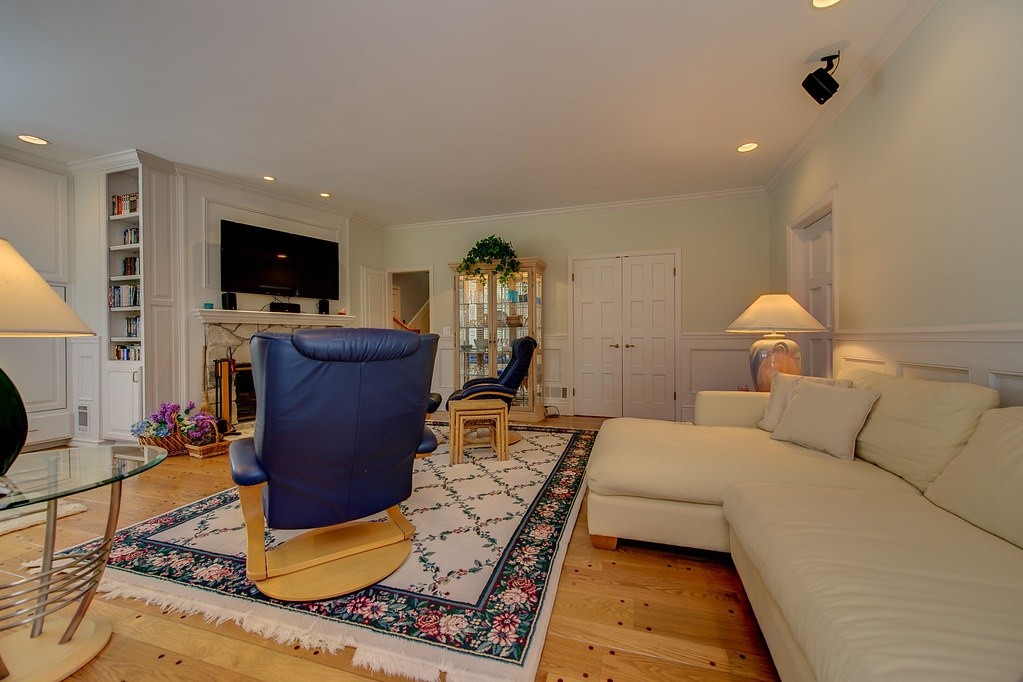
[449,397,509,463]
[0,446,167,682]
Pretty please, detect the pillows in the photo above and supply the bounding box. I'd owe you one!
[760,371,852,429]
[772,378,878,462]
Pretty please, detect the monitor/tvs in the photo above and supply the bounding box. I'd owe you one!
[218,219,339,301]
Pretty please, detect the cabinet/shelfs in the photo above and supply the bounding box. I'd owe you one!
[101,163,145,437]
[449,257,546,422]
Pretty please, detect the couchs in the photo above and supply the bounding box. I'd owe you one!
[585,366,1022,682]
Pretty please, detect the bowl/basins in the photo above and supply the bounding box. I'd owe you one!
[474,339,488,352]
[461,345,472,351]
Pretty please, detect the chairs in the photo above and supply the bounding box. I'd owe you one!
[230,330,441,606]
[445,337,538,449]
[417,392,442,459]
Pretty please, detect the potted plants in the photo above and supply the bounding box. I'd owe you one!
[457,236,520,287]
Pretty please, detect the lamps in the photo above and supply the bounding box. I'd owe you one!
[0,237,96,337]
[725,292,827,389]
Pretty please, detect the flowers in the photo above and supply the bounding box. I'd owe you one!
[130,400,222,442]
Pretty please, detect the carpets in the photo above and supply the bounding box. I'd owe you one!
[24,420,601,681]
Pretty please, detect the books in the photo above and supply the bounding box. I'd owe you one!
[112,192,138,215]
[125,316,140,337]
[122,256,140,276]
[123,228,139,245]
[115,343,140,361]
[109,285,140,308]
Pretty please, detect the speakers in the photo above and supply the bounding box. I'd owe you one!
[222,292,238,310]
[801,68,840,105]
[270,302,301,313]
[318,300,329,314]
